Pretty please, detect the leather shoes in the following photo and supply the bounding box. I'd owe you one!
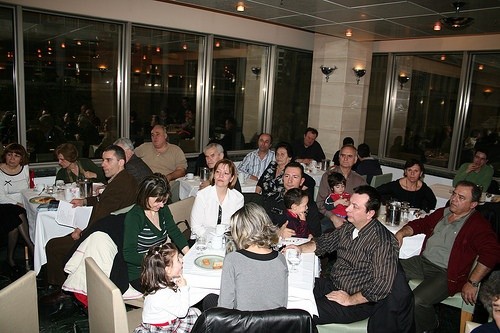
[40,292,73,303]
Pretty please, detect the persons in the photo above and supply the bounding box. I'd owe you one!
[449,148,495,200]
[278,188,313,240]
[388,120,500,172]
[123,172,190,293]
[1,95,246,159]
[135,243,202,333]
[315,146,369,233]
[39,144,138,322]
[236,133,279,181]
[371,159,438,213]
[203,203,289,313]
[286,126,383,185]
[324,171,355,223]
[0,143,40,272]
[261,161,322,242]
[391,180,500,333]
[277,185,400,333]
[467,271,500,333]
[44,121,242,204]
[189,160,245,249]
[254,143,315,205]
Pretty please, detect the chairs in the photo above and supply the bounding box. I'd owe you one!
[83,256,147,333]
[1,268,41,333]
[407,273,482,331]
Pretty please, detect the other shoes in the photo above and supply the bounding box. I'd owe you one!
[8,259,20,273]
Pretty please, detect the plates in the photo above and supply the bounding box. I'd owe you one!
[194,254,225,270]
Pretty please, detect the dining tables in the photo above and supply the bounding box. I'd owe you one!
[20,182,97,276]
[348,203,429,260]
[180,222,323,319]
[288,160,329,202]
[426,182,494,212]
[174,171,259,200]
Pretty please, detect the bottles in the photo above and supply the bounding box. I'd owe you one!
[200,166,209,181]
[300,157,331,173]
[379,200,426,228]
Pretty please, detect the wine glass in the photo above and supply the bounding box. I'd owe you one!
[287,250,302,273]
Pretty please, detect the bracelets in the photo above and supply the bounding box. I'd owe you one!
[466,279,479,287]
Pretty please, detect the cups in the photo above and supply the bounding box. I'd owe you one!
[185,173,193,179]
[193,223,233,255]
[29,177,107,205]
